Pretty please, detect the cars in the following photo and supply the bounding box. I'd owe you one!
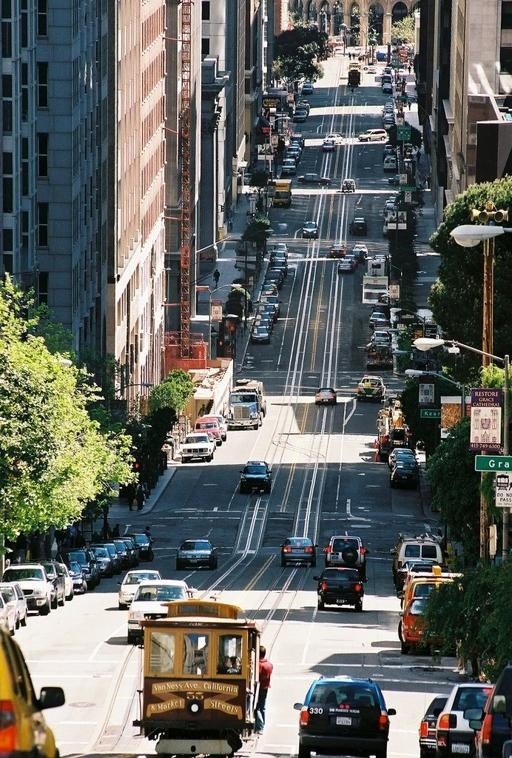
[239,460,273,495]
[0,532,220,646]
[280,537,317,568]
[357,371,419,489]
[315,387,338,406]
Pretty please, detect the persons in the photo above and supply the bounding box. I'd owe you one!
[255,646,273,733]
[190,635,241,674]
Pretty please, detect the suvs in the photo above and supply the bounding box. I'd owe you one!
[0,629,64,758]
[290,662,511,756]
[391,531,468,656]
[324,535,368,582]
[313,564,364,612]
[180,413,227,461]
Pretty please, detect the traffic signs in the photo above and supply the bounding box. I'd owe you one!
[472,446,510,473]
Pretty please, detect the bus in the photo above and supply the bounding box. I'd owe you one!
[133,588,260,756]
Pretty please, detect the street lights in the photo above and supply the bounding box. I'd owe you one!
[443,222,512,251]
[409,334,510,566]
[207,283,243,360]
[404,366,467,419]
[100,378,160,530]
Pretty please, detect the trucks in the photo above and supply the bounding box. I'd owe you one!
[226,376,268,433]
